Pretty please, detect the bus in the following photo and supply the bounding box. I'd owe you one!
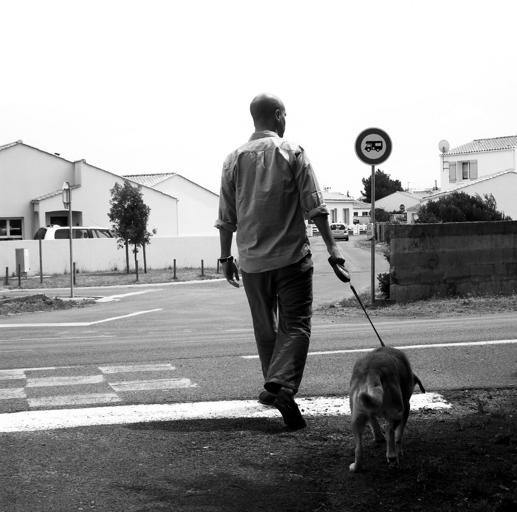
[364,140,383,152]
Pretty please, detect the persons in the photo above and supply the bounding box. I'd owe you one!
[215,93,345,428]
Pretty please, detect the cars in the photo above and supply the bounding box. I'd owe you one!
[328,223,348,242]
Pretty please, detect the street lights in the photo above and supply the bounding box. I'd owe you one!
[61,180,72,299]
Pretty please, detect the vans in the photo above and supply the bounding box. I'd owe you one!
[34,226,113,239]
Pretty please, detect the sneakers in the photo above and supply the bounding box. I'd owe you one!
[258,391,278,405]
[274,392,308,431]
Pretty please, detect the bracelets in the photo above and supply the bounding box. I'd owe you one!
[218,254,234,264]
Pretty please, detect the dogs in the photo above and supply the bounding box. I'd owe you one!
[349,346,427,472]
